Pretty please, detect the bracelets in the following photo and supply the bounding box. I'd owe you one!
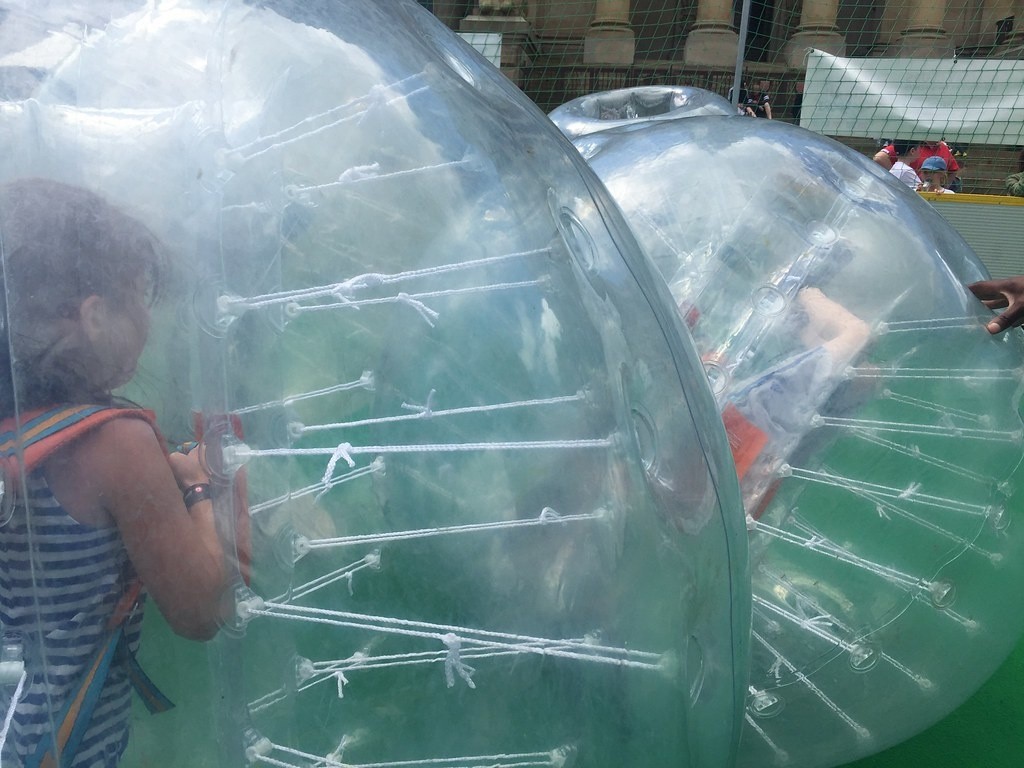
[181,482,212,511]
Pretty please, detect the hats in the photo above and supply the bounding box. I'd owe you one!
[918,156,946,171]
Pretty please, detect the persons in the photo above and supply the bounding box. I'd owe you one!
[643,74,660,86]
[667,248,888,540]
[890,137,925,192]
[745,83,773,119]
[1,188,238,766]
[966,275,1024,333]
[916,157,955,193]
[873,139,959,190]
[729,80,749,116]
[1004,167,1024,196]
